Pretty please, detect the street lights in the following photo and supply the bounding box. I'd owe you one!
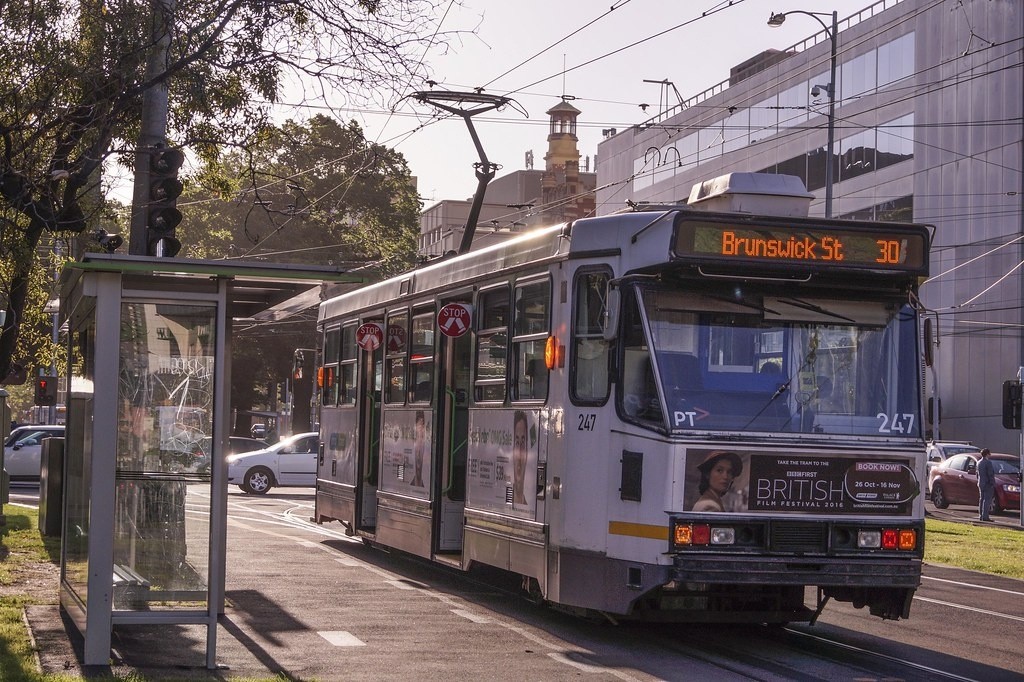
[151,146,183,237]
[768,9,838,217]
[94,232,123,254]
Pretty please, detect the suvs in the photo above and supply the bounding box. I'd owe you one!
[925,440,980,494]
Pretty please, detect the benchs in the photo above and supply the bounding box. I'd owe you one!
[111,559,151,610]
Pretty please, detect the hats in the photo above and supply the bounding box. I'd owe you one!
[697,450,743,478]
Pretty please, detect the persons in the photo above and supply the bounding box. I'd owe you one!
[690,451,743,513]
[410,410,425,487]
[511,411,528,504]
[977,448,995,522]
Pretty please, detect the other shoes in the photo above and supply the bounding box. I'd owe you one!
[984,519,995,522]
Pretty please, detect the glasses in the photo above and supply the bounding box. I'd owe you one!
[988,453,991,455]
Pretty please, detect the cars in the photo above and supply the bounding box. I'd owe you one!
[3,427,67,484]
[927,452,1021,515]
[188,437,272,482]
[227,432,316,495]
[251,424,266,437]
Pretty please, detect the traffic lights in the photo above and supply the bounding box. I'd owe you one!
[277,381,285,402]
[35,376,57,405]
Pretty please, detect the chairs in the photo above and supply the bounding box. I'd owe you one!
[327,350,696,417]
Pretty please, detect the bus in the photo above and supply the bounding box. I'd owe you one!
[30,405,66,424]
[292,172,939,632]
